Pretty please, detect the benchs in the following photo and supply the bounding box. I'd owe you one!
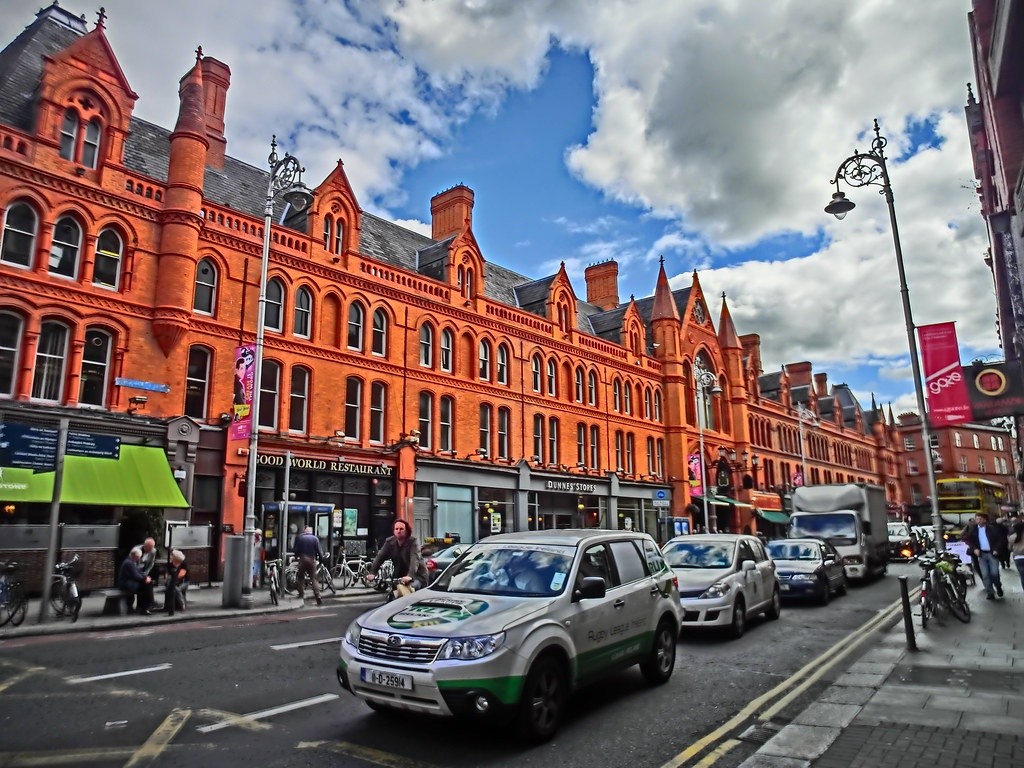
[100,587,187,615]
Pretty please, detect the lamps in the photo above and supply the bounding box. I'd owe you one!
[525,455,539,463]
[76,168,85,176]
[465,301,472,305]
[560,464,568,469]
[441,450,457,455]
[221,413,232,427]
[649,471,657,478]
[568,462,584,470]
[708,444,759,473]
[328,430,345,439]
[127,395,148,415]
[604,468,624,474]
[469,448,487,456]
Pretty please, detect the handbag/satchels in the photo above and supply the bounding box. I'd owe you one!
[397,583,415,597]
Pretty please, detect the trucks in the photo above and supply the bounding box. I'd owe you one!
[785,482,891,587]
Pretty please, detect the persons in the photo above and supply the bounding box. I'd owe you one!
[546,555,585,594]
[233,356,246,404]
[119,547,164,614]
[129,538,156,576]
[366,519,429,597]
[165,549,189,611]
[688,460,696,480]
[961,510,1024,600]
[252,529,262,588]
[295,526,324,607]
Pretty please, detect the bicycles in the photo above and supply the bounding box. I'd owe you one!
[908,546,974,628]
[264,547,414,606]
[50,553,83,624]
[0,560,31,627]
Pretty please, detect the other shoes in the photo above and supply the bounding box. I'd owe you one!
[997,587,1003,596]
[986,593,994,599]
[141,610,151,615]
[317,597,321,604]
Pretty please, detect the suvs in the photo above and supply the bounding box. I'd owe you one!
[336,528,686,747]
[886,521,913,563]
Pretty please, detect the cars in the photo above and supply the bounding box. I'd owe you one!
[424,543,473,586]
[765,539,849,608]
[911,525,937,556]
[660,533,783,639]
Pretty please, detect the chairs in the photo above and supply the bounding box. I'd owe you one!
[578,555,605,578]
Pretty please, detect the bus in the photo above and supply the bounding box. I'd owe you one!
[926,478,1007,541]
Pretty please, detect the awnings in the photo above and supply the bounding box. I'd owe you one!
[715,495,752,507]
[694,495,729,506]
[753,508,789,524]
[0,442,190,510]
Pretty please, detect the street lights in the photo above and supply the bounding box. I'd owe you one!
[824,117,948,563]
[241,133,315,596]
[694,355,723,533]
